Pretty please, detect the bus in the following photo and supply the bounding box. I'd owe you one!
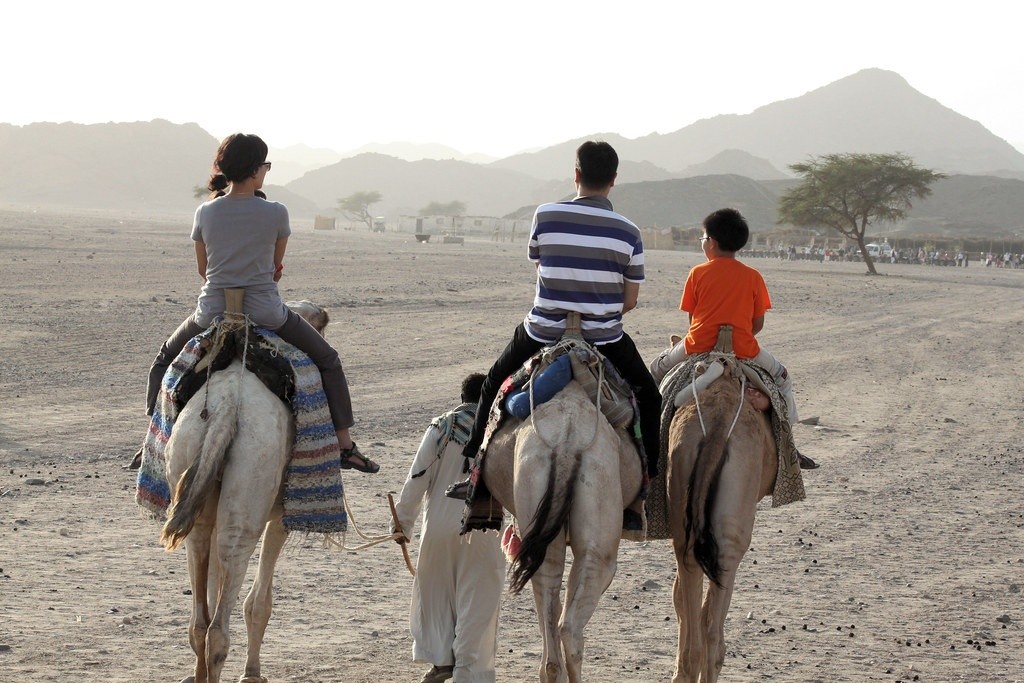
[864,242,892,258]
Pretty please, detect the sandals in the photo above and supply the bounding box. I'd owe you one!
[129,448,142,469]
[340,441,380,474]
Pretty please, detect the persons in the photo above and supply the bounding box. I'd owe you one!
[389,372,508,683]
[444,140,656,532]
[128,132,382,475]
[778,244,970,267]
[650,207,821,470]
[981,252,1023,269]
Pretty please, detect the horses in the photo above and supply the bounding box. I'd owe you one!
[663,331,784,683]
[480,341,645,683]
[163,300,332,683]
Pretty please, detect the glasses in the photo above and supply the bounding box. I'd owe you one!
[699,237,709,243]
[261,161,271,171]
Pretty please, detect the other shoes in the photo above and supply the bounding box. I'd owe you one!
[421,665,453,683]
[445,477,470,499]
[797,449,820,469]
